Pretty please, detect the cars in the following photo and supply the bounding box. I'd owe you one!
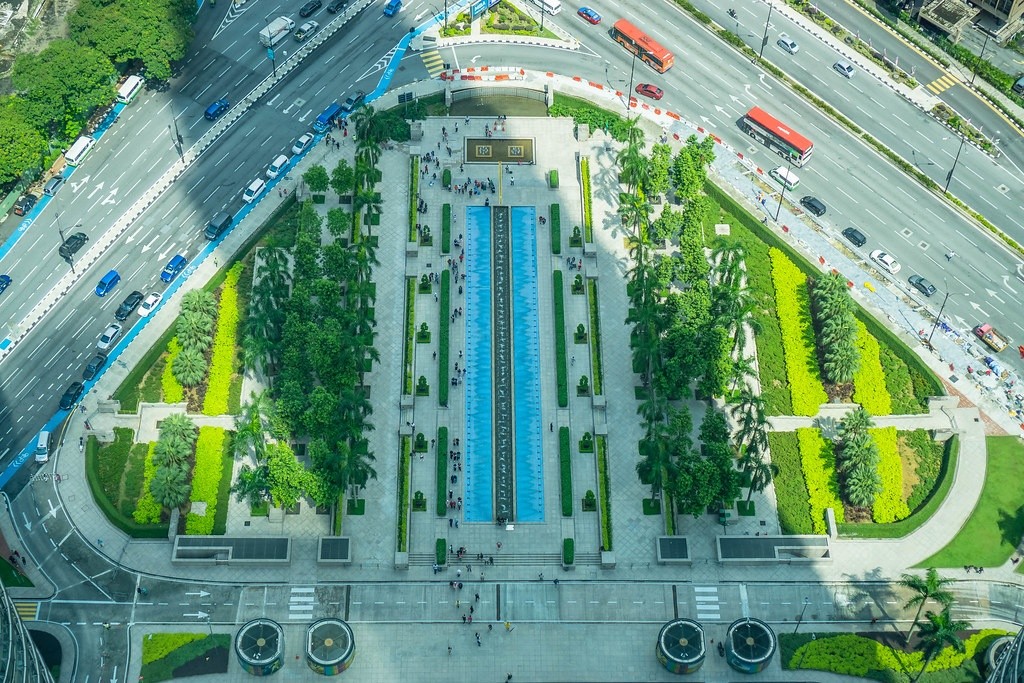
[871,249,901,275]
[908,275,938,298]
[0,274,13,296]
[833,58,856,80]
[635,84,665,100]
[777,35,799,55]
[577,7,602,25]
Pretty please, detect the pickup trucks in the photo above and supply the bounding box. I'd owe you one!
[293,19,321,44]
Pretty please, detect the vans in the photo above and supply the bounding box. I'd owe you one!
[770,164,801,191]
[27,87,367,467]
[532,0,562,15]
[1011,76,1024,94]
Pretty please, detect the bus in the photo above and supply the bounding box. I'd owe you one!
[742,105,815,169]
[612,17,675,74]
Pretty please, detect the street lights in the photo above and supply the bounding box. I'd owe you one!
[926,277,970,343]
[774,165,809,221]
[264,17,290,78]
[170,104,196,163]
[56,213,83,274]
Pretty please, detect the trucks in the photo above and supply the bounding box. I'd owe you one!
[974,321,1011,353]
[259,15,296,47]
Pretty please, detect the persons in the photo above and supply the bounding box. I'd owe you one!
[214,257,218,267]
[948,251,955,260]
[278,187,287,198]
[406,349,466,528]
[319,107,587,323]
[102,622,111,630]
[8,548,27,566]
[79,404,90,453]
[570,356,576,366]
[550,423,554,432]
[135,586,148,595]
[97,539,104,547]
[430,540,515,683]
[30,473,61,483]
[539,573,559,588]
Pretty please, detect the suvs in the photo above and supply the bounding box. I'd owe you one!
[327,0,347,13]
[799,195,826,216]
[841,227,867,247]
[298,0,323,18]
[383,0,402,17]
